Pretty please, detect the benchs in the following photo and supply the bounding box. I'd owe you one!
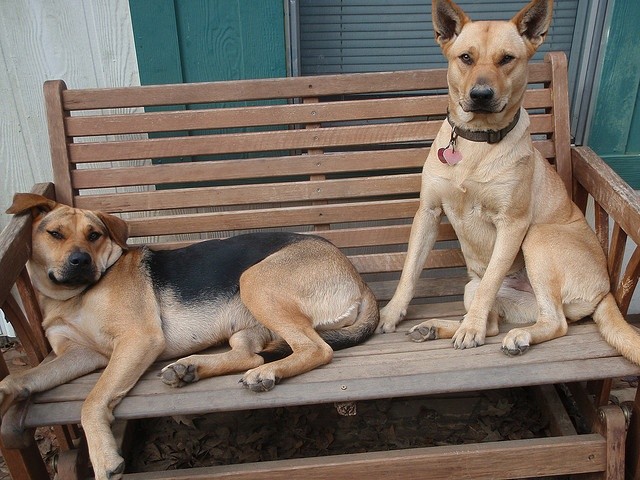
[0,52,640,476]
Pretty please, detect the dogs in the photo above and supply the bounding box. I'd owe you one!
[0,191,381,479]
[373,0,640,368]
